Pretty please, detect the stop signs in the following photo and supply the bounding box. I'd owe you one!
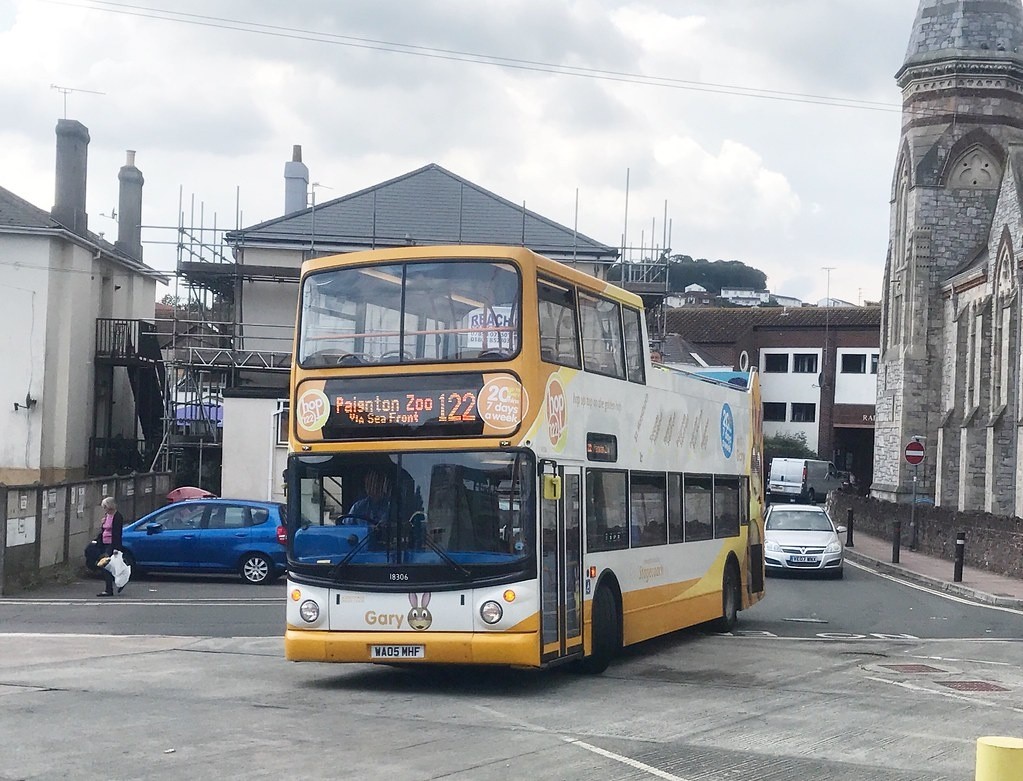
[904,441,925,465]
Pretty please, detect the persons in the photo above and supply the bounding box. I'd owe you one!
[338,470,403,531]
[95,496,128,597]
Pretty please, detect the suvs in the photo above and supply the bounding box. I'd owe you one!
[84,494,311,585]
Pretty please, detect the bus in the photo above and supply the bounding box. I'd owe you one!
[280,246,766,677]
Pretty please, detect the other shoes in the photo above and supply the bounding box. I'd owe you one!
[96,592,113,597]
[117,580,130,594]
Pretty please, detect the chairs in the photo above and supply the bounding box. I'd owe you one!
[209,513,221,528]
[302,346,643,382]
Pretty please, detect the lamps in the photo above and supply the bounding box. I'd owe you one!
[14,394,36,410]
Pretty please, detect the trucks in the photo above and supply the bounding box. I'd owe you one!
[765,457,844,504]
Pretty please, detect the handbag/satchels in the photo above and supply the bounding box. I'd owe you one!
[102,550,131,588]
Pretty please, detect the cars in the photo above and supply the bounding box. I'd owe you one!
[763,503,847,577]
